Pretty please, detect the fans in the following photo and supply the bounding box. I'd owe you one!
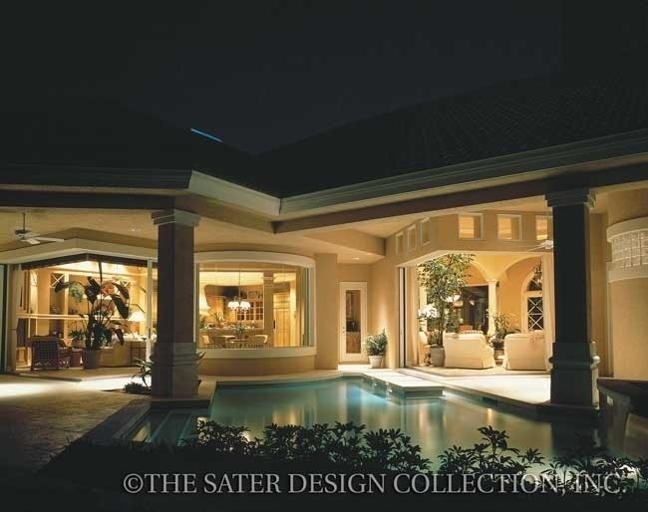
[0,213,64,245]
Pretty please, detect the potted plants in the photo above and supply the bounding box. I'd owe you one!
[54,276,146,368]
[68,329,85,353]
[365,328,388,368]
[486,313,516,348]
[417,254,474,367]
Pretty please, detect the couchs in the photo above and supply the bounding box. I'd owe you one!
[504,333,546,370]
[419,331,435,365]
[94,341,146,367]
[444,335,495,369]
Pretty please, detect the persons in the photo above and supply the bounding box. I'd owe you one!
[72,338,85,352]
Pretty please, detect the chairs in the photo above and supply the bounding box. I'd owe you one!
[200,335,268,348]
[31,337,58,371]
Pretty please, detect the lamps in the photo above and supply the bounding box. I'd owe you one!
[128,311,144,321]
[228,271,250,312]
